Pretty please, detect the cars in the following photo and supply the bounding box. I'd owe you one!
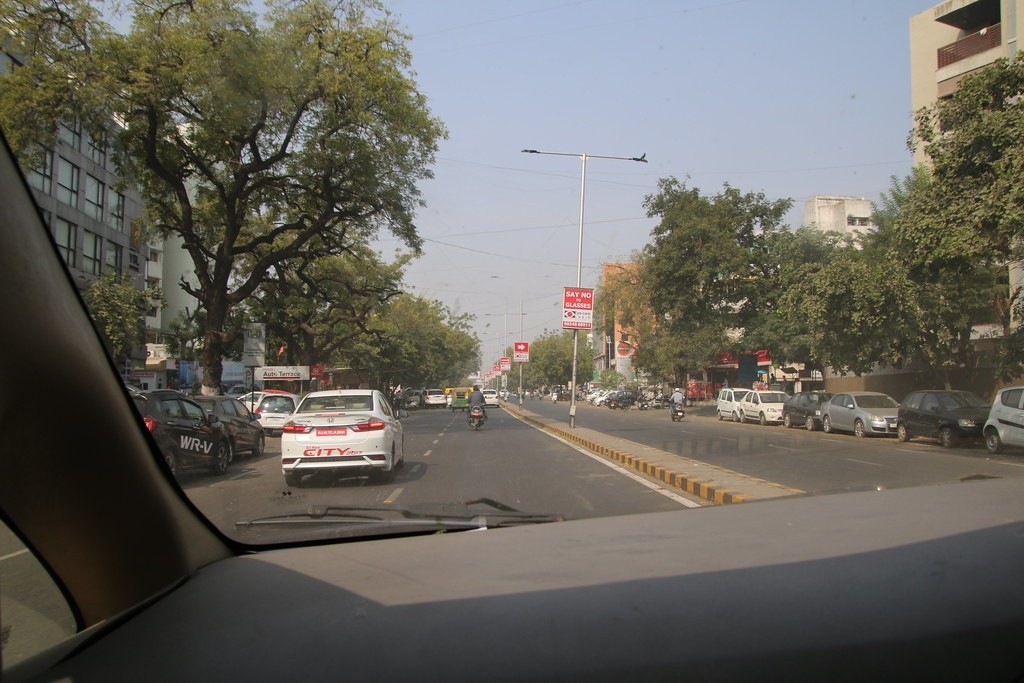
[782,390,835,431]
[897,389,991,448]
[281,389,409,486]
[237,391,302,435]
[983,386,1024,453]
[188,396,266,462]
[820,391,900,438]
[739,390,791,425]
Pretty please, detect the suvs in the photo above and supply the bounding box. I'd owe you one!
[399,387,510,408]
[128,389,230,478]
[586,388,639,406]
[717,387,751,422]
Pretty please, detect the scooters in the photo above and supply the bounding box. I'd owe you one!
[467,396,488,430]
[607,394,648,411]
[552,392,558,403]
[670,399,685,423]
[446,395,453,410]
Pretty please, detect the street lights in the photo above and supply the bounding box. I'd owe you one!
[482,312,528,401]
[522,148,648,428]
[490,274,553,407]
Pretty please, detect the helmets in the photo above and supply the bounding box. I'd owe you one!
[473,385,480,390]
[675,388,679,392]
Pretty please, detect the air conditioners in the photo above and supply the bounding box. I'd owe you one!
[147,350,154,357]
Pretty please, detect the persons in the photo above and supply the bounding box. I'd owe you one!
[720,376,728,388]
[783,376,788,392]
[770,373,776,383]
[168,375,176,389]
[637,388,642,409]
[395,391,403,398]
[552,391,557,401]
[467,385,488,420]
[445,390,452,398]
[669,388,685,415]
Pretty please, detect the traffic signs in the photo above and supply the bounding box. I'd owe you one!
[514,341,529,362]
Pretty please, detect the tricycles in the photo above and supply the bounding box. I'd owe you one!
[452,387,471,412]
